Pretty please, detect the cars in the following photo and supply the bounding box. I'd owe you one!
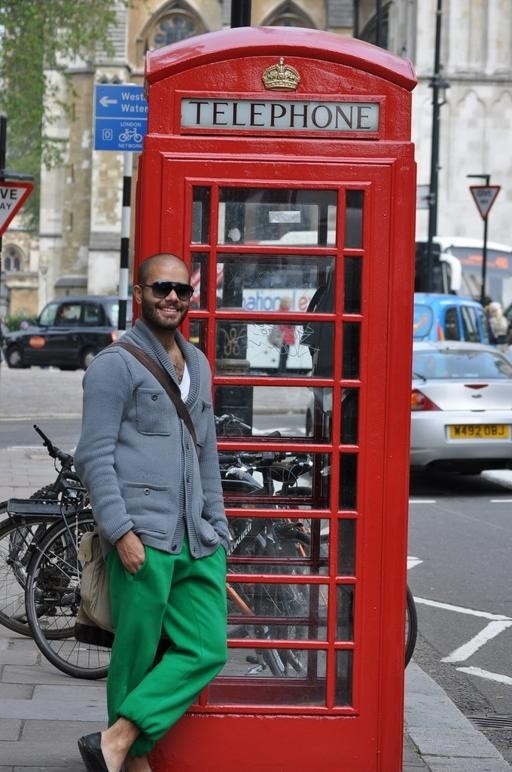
[409,340,512,488]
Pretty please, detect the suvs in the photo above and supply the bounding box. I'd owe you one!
[2,293,133,372]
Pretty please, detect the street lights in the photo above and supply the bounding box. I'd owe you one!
[426,71,451,295]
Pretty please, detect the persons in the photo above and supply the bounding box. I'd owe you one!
[72,250,232,772]
[274,298,296,373]
[482,298,490,312]
[489,302,512,355]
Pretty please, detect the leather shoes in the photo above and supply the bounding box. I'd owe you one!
[77,731,109,770]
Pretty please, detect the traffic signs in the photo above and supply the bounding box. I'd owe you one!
[92,85,151,152]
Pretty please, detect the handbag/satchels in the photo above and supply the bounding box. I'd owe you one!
[74,532,118,630]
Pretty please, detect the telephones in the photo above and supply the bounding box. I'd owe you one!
[299,256,360,378]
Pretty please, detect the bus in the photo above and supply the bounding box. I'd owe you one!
[189,233,512,376]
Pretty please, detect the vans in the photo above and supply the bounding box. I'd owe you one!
[413,291,500,379]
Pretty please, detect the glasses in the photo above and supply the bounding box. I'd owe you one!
[136,280,194,300]
